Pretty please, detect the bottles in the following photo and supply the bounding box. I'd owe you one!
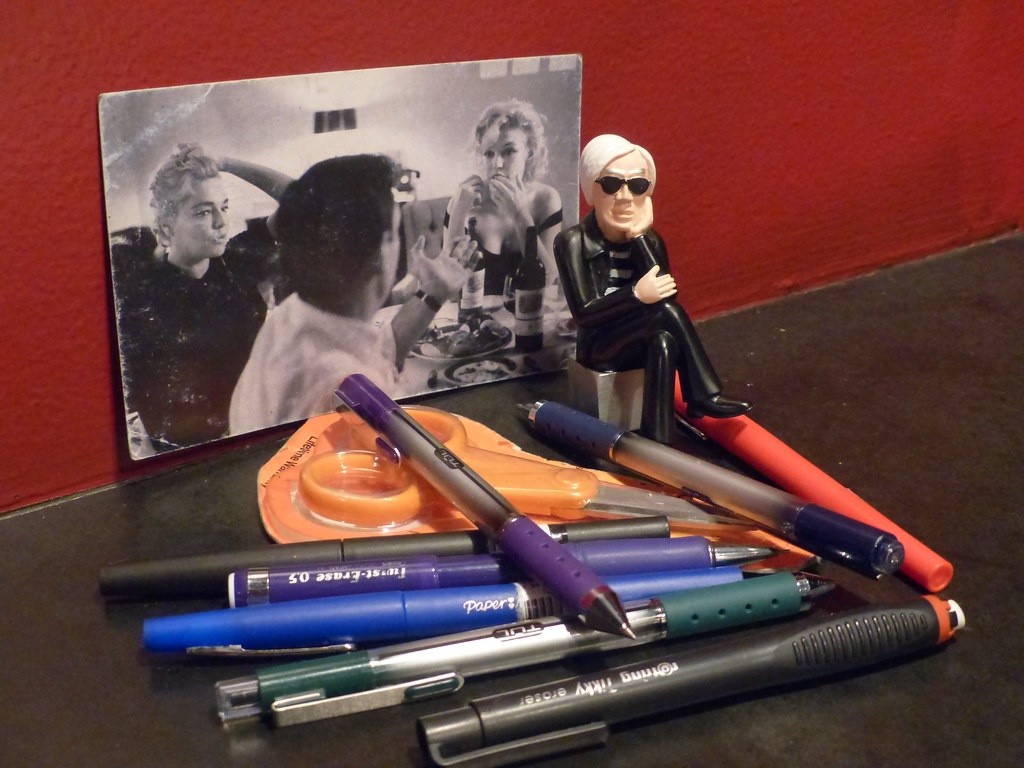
[458,217,484,314]
[514,227,547,353]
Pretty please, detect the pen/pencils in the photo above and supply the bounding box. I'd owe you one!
[335,371,637,641]
[514,401,906,578]
[98,515,968,768]
[672,369,955,593]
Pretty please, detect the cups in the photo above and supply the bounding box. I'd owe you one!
[503,275,515,314]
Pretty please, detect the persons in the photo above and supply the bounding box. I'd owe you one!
[115,140,297,454]
[442,98,566,295]
[229,154,485,434]
[554,134,755,452]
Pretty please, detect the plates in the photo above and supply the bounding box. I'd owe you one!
[409,320,513,366]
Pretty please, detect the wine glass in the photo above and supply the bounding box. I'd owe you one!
[544,277,568,330]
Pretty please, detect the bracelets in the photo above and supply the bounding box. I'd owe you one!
[413,289,442,312]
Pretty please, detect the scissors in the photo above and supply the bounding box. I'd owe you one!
[296,406,764,530]
